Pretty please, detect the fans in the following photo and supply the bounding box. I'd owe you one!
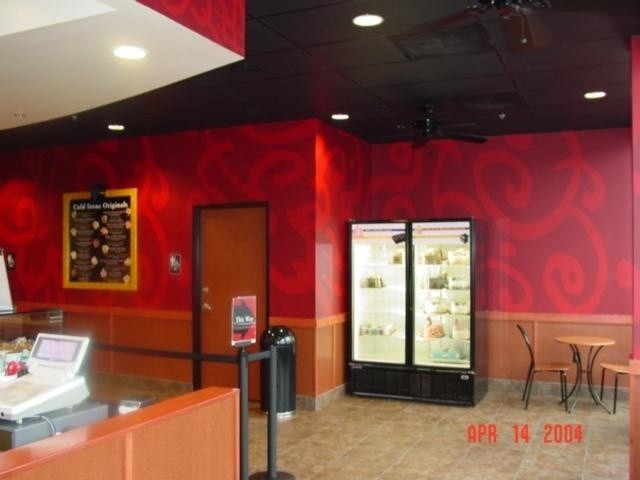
[386,1,590,51]
[364,99,488,150]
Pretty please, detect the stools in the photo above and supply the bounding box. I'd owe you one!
[599,362,628,412]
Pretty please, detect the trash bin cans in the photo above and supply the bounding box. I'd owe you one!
[261,326,296,420]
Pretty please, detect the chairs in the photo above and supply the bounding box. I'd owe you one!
[555,336,615,412]
[516,324,573,412]
